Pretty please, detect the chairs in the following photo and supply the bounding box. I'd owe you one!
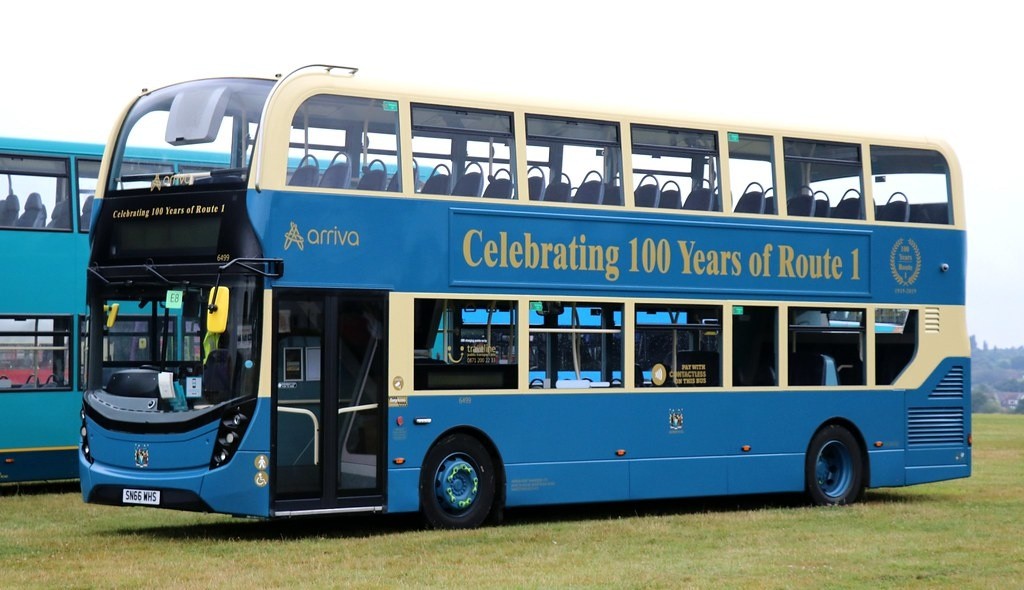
[569,171,625,208]
[878,192,912,224]
[80,196,95,228]
[320,151,352,189]
[833,190,878,221]
[483,169,512,200]
[659,182,682,210]
[46,198,72,230]
[387,157,419,194]
[421,165,452,196]
[289,155,320,188]
[527,168,547,202]
[734,183,774,217]
[16,193,47,229]
[0,196,20,227]
[543,174,572,205]
[530,378,679,388]
[634,175,660,208]
[683,177,733,213]
[452,161,485,198]
[787,186,831,219]
[357,159,387,191]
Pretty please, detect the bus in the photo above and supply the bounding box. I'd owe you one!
[0,343,68,384]
[434,298,833,384]
[78,64,974,530]
[0,137,439,480]
